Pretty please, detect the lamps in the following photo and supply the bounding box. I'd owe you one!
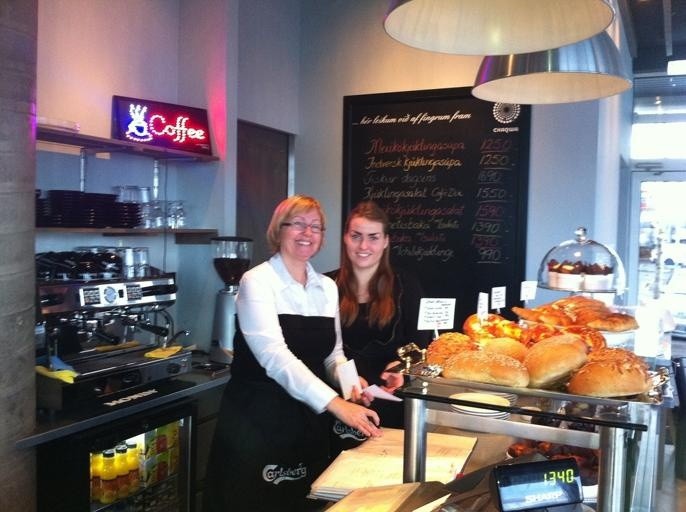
[472,32,633,106]
[383,0,615,55]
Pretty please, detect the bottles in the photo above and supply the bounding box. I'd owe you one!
[89,441,140,503]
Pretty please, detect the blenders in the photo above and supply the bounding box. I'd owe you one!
[208,237,253,366]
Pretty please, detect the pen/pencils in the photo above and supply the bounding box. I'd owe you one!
[357,400,373,421]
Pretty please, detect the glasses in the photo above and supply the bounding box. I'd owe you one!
[281,221,326,233]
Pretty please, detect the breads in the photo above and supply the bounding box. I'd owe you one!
[425,296,654,398]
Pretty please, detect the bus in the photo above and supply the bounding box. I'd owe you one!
[661,236,686,268]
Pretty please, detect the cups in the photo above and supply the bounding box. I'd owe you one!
[74,247,151,278]
[113,185,187,229]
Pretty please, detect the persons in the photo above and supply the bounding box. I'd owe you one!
[206,195,381,512]
[323,199,424,429]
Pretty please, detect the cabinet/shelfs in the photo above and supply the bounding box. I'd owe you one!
[35,127,221,234]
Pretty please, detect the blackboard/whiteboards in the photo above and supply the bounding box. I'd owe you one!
[341,86,532,350]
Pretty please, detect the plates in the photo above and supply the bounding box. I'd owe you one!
[35,189,143,229]
[448,388,518,421]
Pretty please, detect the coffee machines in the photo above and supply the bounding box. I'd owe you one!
[36,249,193,410]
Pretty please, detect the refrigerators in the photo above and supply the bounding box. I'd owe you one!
[37,398,198,512]
